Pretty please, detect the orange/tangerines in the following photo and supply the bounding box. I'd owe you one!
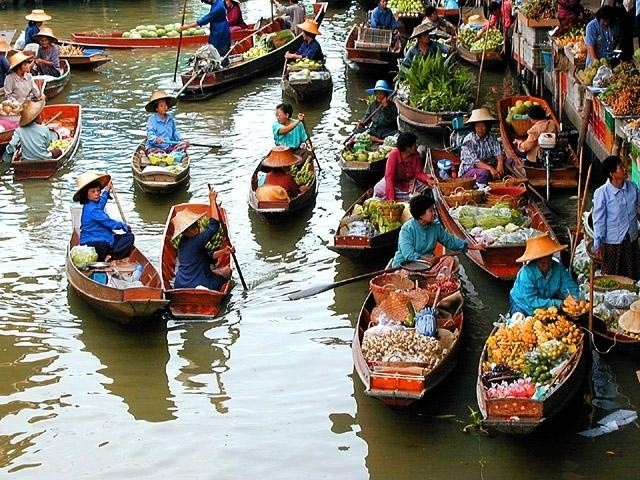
[520,351,567,386]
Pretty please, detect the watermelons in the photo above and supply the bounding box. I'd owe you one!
[121,24,207,39]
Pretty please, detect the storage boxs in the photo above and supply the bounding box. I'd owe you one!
[512,17,552,74]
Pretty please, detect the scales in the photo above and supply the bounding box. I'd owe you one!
[399,259,431,282]
[88,262,113,285]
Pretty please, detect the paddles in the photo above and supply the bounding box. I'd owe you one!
[288,264,402,301]
[506,164,559,215]
[164,140,220,148]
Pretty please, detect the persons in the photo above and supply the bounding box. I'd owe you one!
[508,233,580,319]
[359,80,399,144]
[482,2,516,40]
[586,0,640,84]
[262,146,309,199]
[591,155,639,281]
[513,105,558,168]
[421,6,456,38]
[145,90,190,159]
[5,100,62,160]
[170,187,236,291]
[403,24,449,68]
[0,9,61,105]
[181,0,323,68]
[371,1,400,34]
[557,1,584,36]
[392,195,487,268]
[71,171,135,261]
[374,132,438,201]
[257,104,313,186]
[458,109,506,190]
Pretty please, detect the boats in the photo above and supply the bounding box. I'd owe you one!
[163,203,234,321]
[247,145,317,228]
[175,2,330,100]
[0,30,110,182]
[54,21,262,48]
[282,52,333,107]
[352,259,465,409]
[129,138,191,194]
[476,309,585,439]
[64,231,167,325]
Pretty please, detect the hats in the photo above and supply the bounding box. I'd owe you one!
[516,235,569,263]
[619,300,640,333]
[365,80,393,96]
[262,147,301,168]
[296,20,322,37]
[0,10,59,71]
[464,109,497,124]
[19,99,46,124]
[171,206,208,241]
[73,172,111,203]
[145,91,177,111]
[409,24,437,39]
[465,15,490,29]
